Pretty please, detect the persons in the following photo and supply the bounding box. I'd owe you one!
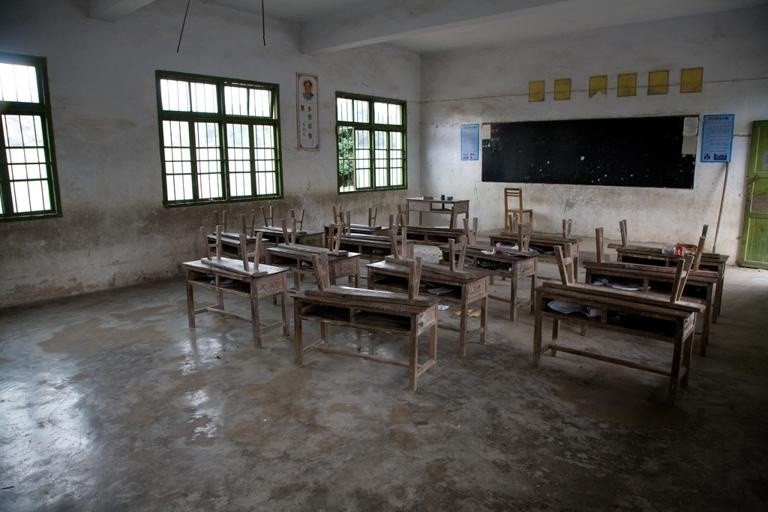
[302,79,314,100]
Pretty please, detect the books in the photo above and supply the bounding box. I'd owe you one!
[592,273,648,292]
[538,293,602,324]
[491,239,553,255]
[441,250,513,273]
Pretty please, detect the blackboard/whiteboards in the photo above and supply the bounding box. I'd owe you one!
[481,114,699,189]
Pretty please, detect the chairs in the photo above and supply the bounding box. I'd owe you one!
[503,186,534,231]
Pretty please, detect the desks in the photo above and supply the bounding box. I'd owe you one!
[181,196,729,410]
